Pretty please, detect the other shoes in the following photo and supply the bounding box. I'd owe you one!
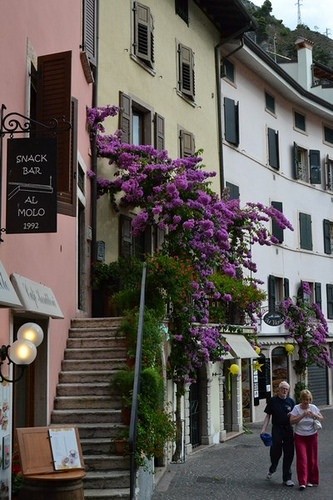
[307,483,313,487]
[299,485,307,489]
[283,480,295,487]
[265,472,273,481]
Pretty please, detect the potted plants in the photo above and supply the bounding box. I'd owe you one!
[94,259,177,474]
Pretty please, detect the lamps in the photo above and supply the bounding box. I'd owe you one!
[0,322,44,383]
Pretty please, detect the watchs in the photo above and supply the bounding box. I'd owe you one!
[314,416,316,420]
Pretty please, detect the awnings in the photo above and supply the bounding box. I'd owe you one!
[0,262,65,319]
[207,335,261,360]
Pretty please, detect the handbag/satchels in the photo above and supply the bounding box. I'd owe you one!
[312,420,322,430]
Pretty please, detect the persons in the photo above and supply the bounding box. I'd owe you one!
[288,389,324,489]
[260,381,296,486]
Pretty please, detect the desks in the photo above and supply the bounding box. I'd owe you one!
[22,470,86,500]
